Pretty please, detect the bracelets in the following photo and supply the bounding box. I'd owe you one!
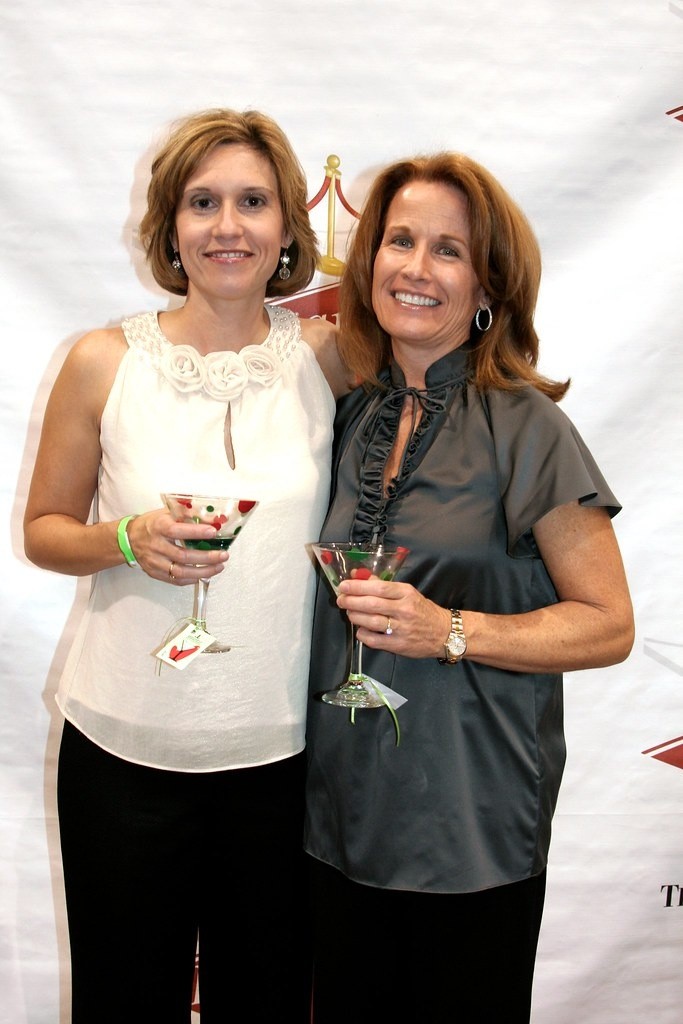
[117,515,145,570]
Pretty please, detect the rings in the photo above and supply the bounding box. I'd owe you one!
[385,616,393,634]
[168,561,176,578]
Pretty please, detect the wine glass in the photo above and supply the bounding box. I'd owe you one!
[309,543,409,708]
[157,493,260,655]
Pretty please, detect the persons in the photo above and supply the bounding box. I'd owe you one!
[21,104,374,1023]
[298,151,637,1024]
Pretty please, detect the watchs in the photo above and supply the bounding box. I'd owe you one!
[445,609,468,663]
